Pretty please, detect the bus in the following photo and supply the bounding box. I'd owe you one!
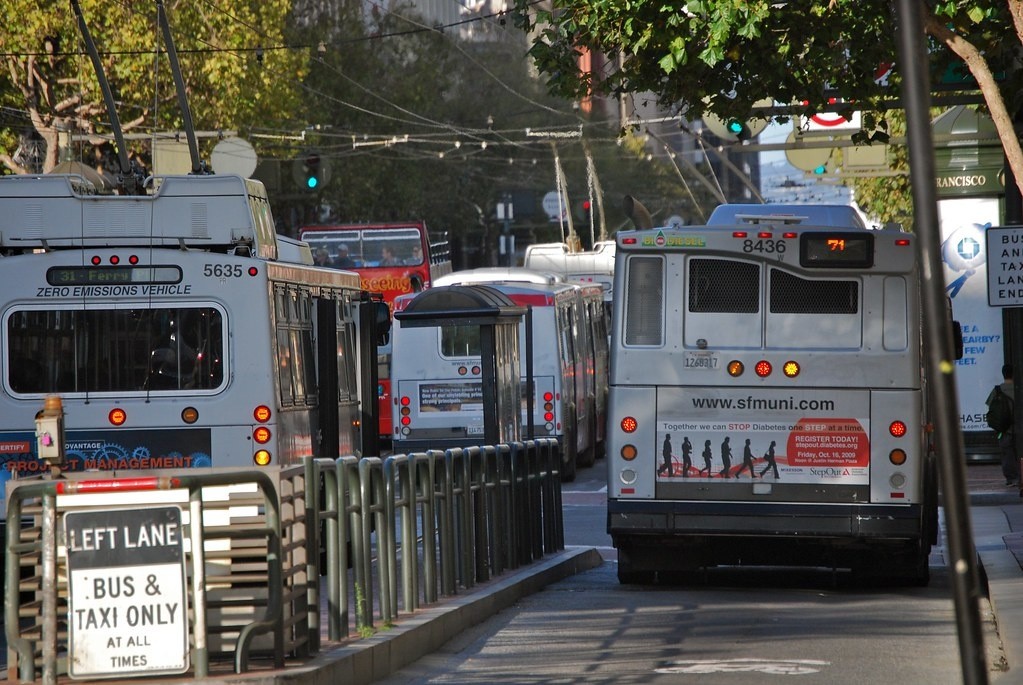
[0,3,966,667]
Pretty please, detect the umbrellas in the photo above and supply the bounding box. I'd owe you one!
[39,129,116,195]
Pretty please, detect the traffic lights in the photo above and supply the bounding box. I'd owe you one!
[699,69,772,142]
[291,151,331,193]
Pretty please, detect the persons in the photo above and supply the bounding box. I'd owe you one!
[986,364,1023,487]
[314,242,425,266]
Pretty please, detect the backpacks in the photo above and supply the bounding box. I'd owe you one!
[986,386,1014,433]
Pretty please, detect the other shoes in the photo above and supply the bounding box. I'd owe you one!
[1006,481,1020,486]
